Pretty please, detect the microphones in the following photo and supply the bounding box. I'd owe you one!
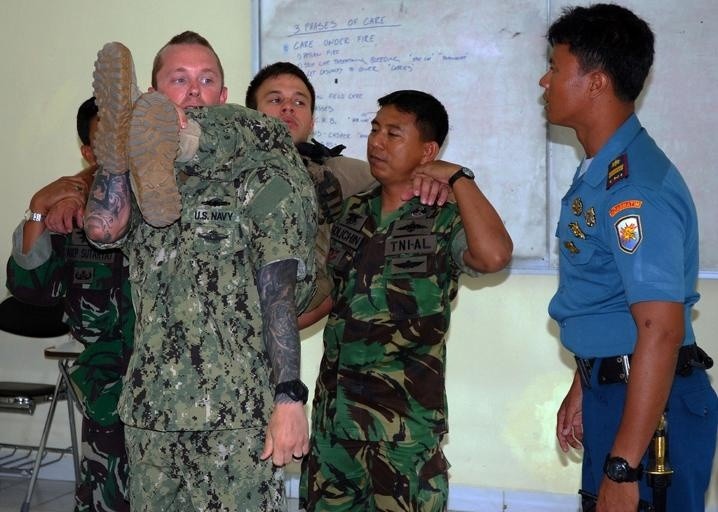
[18,339,88,511]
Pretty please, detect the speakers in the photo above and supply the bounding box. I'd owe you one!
[94,41,143,175]
[128,92,201,229]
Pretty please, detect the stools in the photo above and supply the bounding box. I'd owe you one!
[574,343,714,387]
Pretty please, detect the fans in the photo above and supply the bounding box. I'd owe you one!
[251,1,717,279]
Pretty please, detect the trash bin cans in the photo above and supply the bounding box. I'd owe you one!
[21,209,48,226]
[449,166,474,188]
[601,455,645,483]
[274,379,310,403]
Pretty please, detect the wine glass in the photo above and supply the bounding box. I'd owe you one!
[292,455,302,459]
[75,184,81,191]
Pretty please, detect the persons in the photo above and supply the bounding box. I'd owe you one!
[9,96,136,512]
[95,40,449,317]
[84,30,312,512]
[538,3,718,511]
[294,89,513,511]
